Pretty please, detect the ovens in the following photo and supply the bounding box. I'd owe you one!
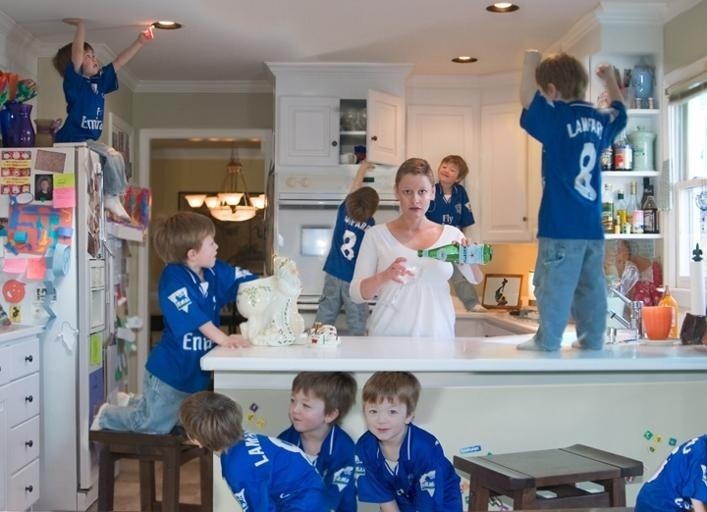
[277,193,402,311]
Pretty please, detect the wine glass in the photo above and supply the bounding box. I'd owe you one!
[339,104,366,132]
[376,260,421,313]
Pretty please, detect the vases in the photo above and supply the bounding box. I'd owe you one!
[14,103,37,148]
[1,100,22,146]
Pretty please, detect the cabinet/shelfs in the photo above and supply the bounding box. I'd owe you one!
[471,68,537,244]
[264,59,409,168]
[1,320,44,512]
[587,0,667,240]
[404,72,481,245]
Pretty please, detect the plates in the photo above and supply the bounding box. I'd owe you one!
[640,339,678,347]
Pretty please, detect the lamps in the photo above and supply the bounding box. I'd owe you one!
[184,144,271,222]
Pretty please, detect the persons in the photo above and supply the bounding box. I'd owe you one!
[52,17,154,222]
[313,160,379,337]
[425,155,486,313]
[36,179,52,200]
[355,372,513,512]
[278,371,358,512]
[517,49,628,352]
[178,390,330,512]
[634,434,707,512]
[90,211,259,436]
[348,158,484,337]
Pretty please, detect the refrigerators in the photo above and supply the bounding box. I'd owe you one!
[2,143,114,510]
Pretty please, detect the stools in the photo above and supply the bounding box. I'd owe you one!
[452,440,644,512]
[88,425,215,512]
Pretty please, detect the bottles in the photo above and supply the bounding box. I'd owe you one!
[30,118,53,148]
[417,242,492,266]
[600,176,659,235]
[656,283,677,336]
[600,126,633,172]
[633,95,654,110]
[8,102,34,147]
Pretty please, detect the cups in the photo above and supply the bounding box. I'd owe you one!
[629,64,653,109]
[353,145,366,163]
[606,327,616,344]
[339,153,355,164]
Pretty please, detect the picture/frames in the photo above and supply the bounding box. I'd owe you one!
[107,112,137,187]
[176,191,267,274]
[481,273,524,309]
